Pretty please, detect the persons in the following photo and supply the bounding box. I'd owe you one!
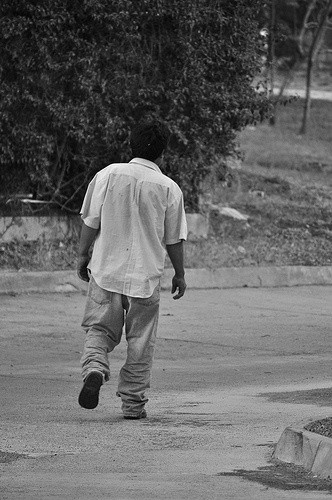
[74,119,186,419]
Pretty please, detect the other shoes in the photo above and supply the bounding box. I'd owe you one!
[125,409,147,419]
[79,371,103,410]
